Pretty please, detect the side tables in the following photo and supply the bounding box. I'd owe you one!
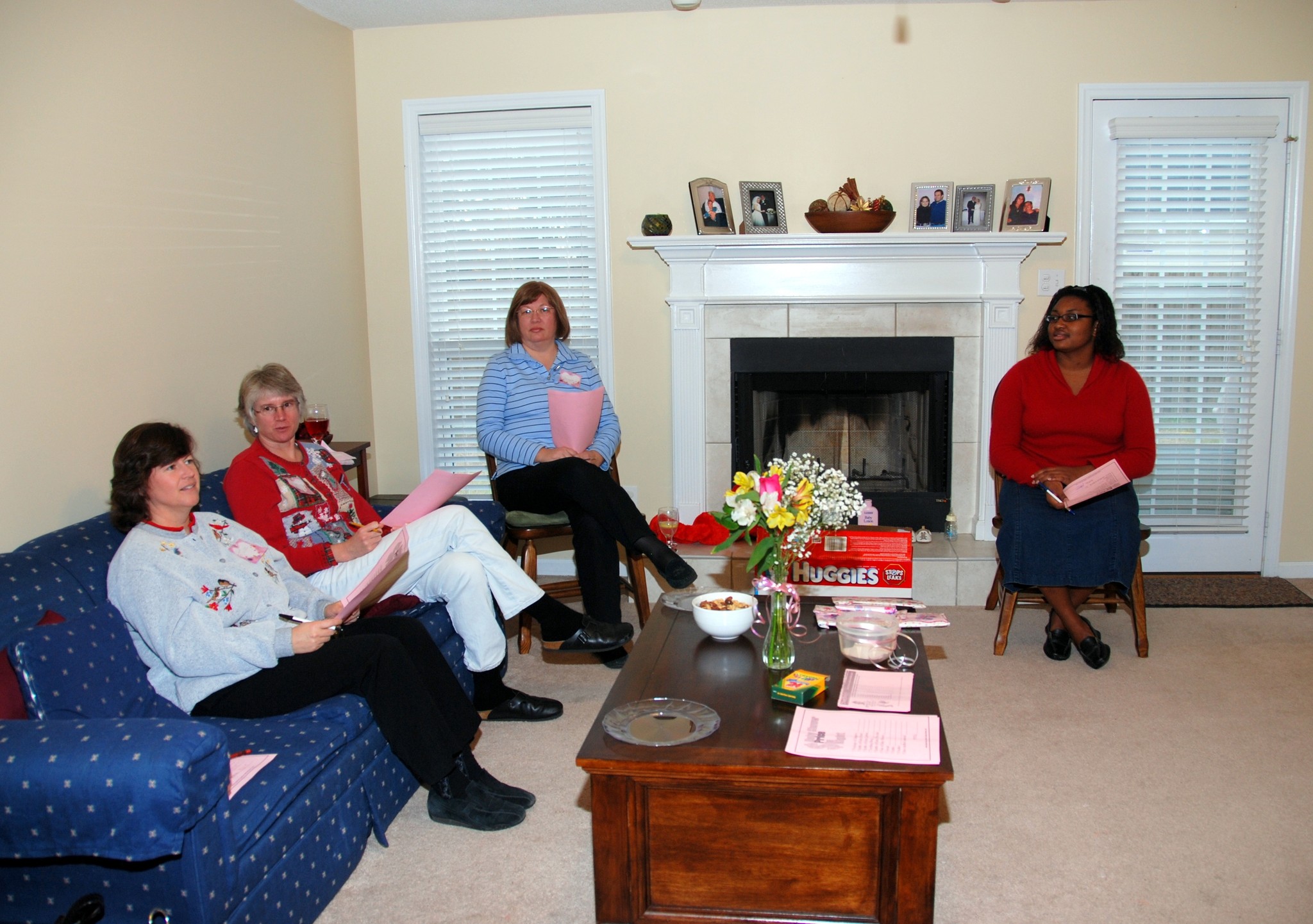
[326,441,371,505]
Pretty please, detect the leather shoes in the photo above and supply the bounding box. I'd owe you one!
[1070,614,1111,669]
[1043,609,1071,661]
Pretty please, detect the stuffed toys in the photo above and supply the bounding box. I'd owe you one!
[704,207,717,218]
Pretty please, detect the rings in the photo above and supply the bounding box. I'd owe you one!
[357,616,360,618]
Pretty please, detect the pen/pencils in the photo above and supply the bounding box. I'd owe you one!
[1038,482,1075,515]
[230,749,252,758]
[349,521,382,533]
[278,614,342,631]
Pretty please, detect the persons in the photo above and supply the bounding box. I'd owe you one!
[991,284,1158,670]
[915,189,1039,226]
[476,282,698,669]
[111,422,537,831]
[221,363,636,722]
[703,191,770,228]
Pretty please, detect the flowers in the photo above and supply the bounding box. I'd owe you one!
[708,451,866,667]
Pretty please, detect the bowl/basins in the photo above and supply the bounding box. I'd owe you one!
[804,210,896,233]
[692,592,758,639]
[836,610,899,664]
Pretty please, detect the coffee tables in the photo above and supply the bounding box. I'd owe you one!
[575,594,954,924]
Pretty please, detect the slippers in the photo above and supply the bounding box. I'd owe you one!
[541,613,634,653]
[476,690,563,722]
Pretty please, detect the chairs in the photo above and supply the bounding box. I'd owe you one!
[986,465,1151,659]
[486,442,650,654]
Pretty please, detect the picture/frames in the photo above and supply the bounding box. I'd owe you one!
[909,181,953,232]
[688,178,736,235]
[739,181,788,234]
[1000,177,1051,231]
[953,184,995,232]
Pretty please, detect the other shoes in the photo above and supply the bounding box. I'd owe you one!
[591,646,628,669]
[427,779,526,831]
[653,546,698,589]
[467,768,535,809]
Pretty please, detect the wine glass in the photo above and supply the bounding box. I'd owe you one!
[658,507,680,553]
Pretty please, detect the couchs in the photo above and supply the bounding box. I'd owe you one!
[0,467,504,924]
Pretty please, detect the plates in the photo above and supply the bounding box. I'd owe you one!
[602,697,721,746]
[659,586,736,611]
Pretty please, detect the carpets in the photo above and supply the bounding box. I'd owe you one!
[1143,577,1313,608]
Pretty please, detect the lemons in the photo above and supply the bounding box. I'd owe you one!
[660,522,678,528]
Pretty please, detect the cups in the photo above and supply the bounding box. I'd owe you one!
[304,404,329,441]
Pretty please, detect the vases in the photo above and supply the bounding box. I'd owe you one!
[761,570,795,669]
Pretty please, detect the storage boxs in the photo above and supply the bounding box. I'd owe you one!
[770,667,831,704]
[755,525,912,598]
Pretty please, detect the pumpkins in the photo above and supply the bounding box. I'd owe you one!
[827,191,851,211]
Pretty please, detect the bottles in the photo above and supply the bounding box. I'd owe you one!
[945,510,957,541]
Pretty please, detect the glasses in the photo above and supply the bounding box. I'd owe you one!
[251,396,300,416]
[516,307,555,318]
[1045,312,1094,324]
